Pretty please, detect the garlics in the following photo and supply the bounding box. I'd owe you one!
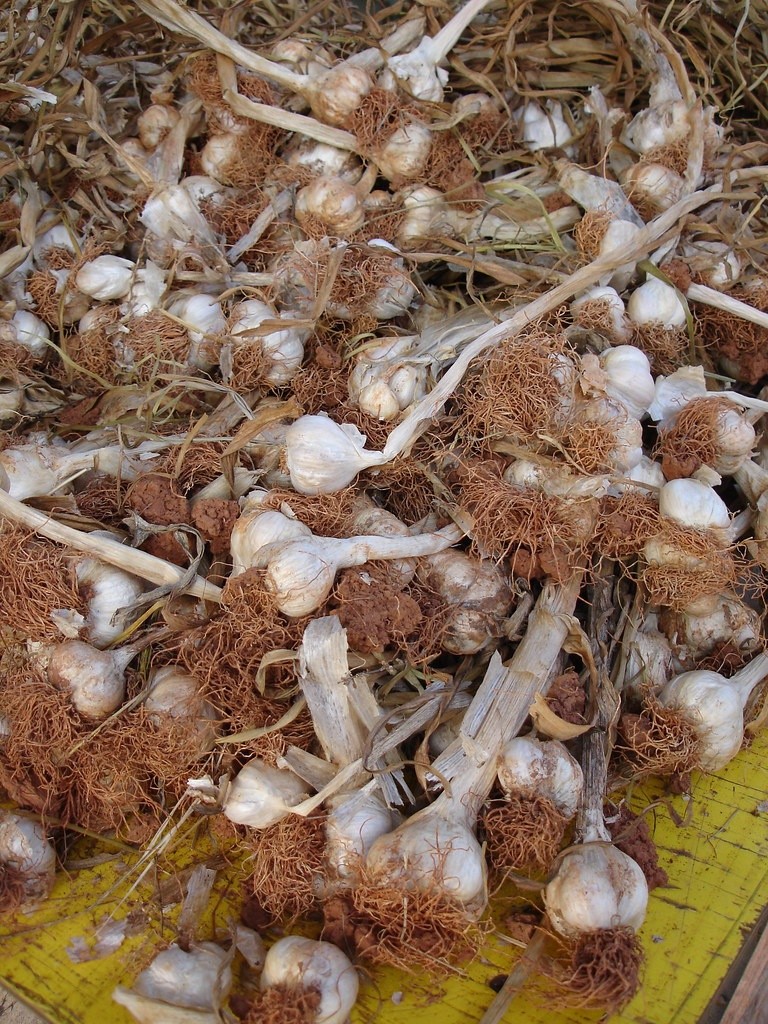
[0,34,768,1024]
